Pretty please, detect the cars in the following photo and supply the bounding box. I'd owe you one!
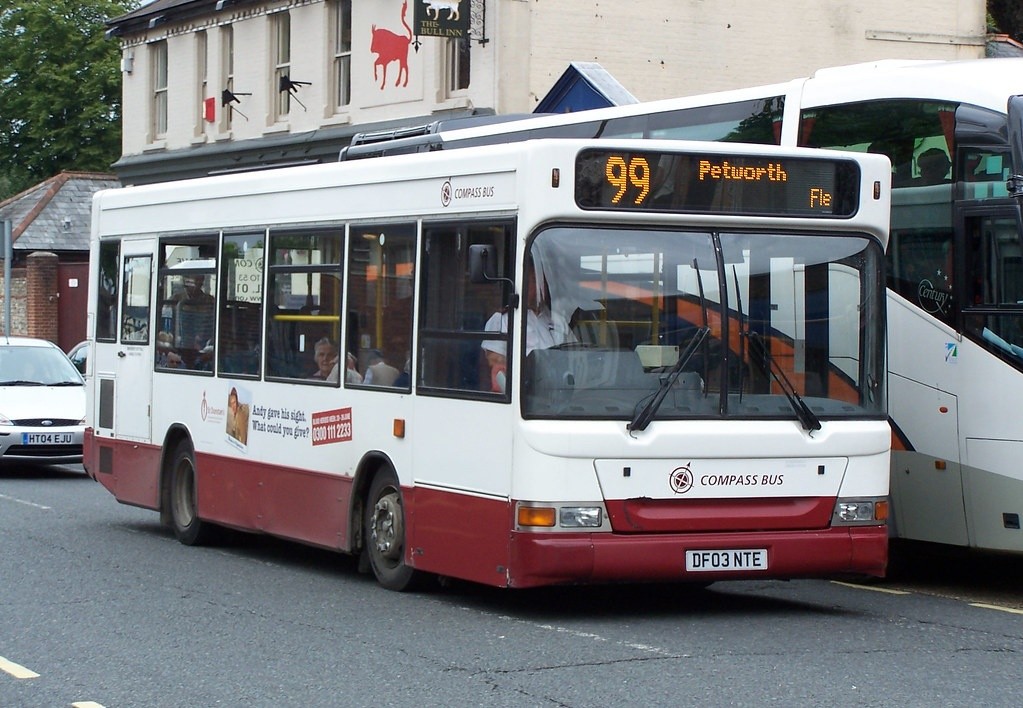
[0,335,84,468]
[66,341,88,378]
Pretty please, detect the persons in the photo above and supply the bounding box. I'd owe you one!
[481,258,577,392]
[916,149,950,187]
[868,141,899,182]
[163,271,408,386]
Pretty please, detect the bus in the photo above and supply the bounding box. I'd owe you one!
[344,55,1023,586]
[84,136,895,594]
